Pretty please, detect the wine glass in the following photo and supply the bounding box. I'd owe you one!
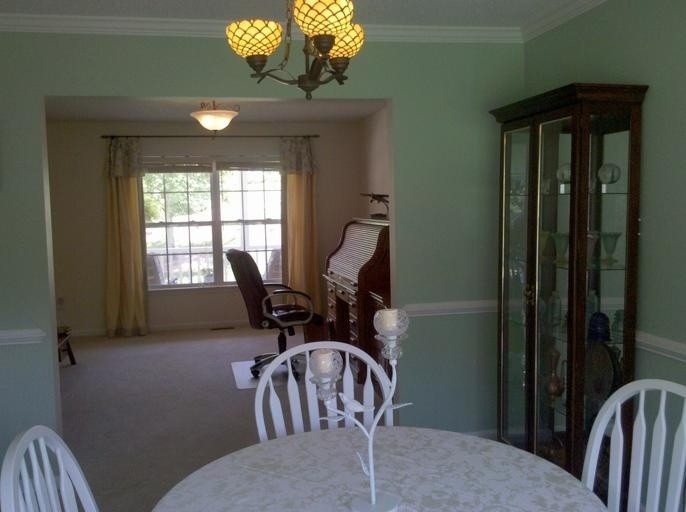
[549,231,569,265]
[597,230,621,269]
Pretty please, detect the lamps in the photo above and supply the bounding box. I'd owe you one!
[189,100,240,139]
[225,0,365,101]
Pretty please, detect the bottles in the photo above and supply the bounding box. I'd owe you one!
[545,289,563,338]
[584,288,600,340]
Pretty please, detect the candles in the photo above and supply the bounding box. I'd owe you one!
[318,349,334,372]
[380,302,398,331]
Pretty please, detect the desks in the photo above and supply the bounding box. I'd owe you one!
[152,427,608,511]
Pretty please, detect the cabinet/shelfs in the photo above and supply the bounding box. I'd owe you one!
[488,83,650,494]
[323,216,389,383]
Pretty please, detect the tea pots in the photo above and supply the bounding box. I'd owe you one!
[544,370,566,396]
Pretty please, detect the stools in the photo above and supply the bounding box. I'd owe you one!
[58,328,76,365]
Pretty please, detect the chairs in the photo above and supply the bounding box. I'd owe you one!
[254,340,393,442]
[228,249,321,382]
[0,422,99,512]
[581,379,686,512]
